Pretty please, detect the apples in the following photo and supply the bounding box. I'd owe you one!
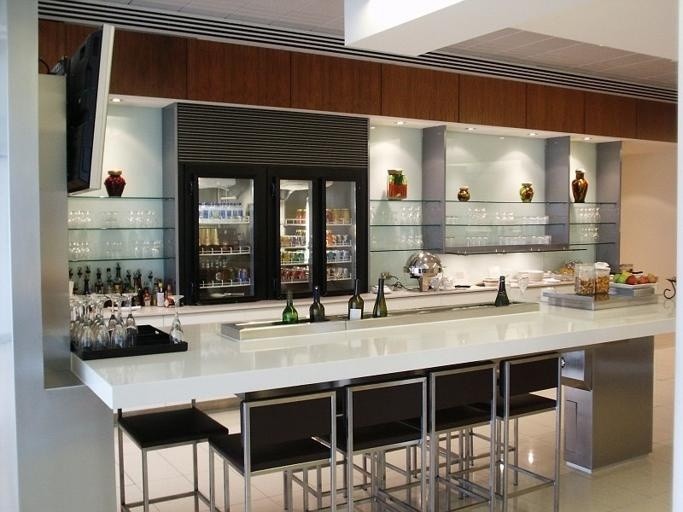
[626,275,637,285]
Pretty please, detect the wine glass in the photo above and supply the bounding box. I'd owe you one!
[572,208,602,243]
[69,292,137,349]
[165,294,184,346]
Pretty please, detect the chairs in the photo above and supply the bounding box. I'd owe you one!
[283,376,431,512]
[460,351,559,510]
[105,342,230,511]
[206,389,339,511]
[375,359,499,510]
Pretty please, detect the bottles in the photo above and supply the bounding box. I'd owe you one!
[347,277,364,320]
[68,263,172,306]
[283,292,299,323]
[571,261,610,296]
[198,258,235,283]
[373,278,387,318]
[308,285,325,322]
[295,208,306,224]
[495,276,509,307]
[326,208,350,224]
[199,200,244,223]
[199,226,246,253]
[563,263,573,281]
[105,168,126,197]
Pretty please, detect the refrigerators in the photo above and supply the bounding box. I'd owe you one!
[159,103,368,300]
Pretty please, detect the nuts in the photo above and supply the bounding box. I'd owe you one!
[575,276,609,295]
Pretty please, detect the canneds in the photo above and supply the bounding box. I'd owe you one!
[280,209,352,281]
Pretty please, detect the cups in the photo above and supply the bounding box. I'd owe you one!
[369,207,423,249]
[431,278,454,294]
[444,206,548,246]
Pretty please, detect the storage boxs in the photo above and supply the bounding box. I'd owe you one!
[575,263,609,295]
[609,280,658,297]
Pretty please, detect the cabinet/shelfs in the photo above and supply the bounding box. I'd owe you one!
[568,202,616,245]
[369,199,442,252]
[446,199,568,254]
[66,193,174,262]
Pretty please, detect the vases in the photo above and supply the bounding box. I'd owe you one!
[105,173,126,198]
[519,182,534,202]
[458,187,470,202]
[572,170,588,203]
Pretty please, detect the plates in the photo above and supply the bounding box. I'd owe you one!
[609,281,657,288]
[481,269,561,285]
